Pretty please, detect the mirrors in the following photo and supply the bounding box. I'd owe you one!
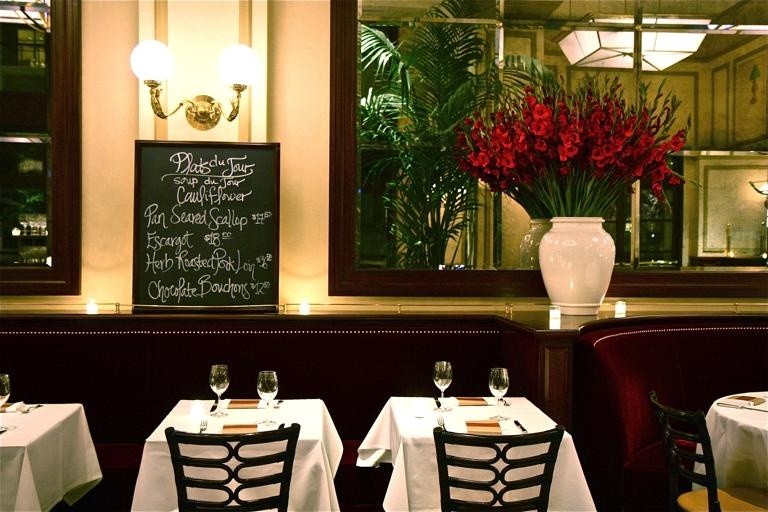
[0,1,84,295]
[326,0,767,297]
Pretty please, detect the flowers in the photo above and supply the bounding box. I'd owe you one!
[462,69,703,216]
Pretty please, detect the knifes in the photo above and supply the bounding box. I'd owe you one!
[513,419,526,432]
[209,398,218,412]
[717,402,768,413]
[433,395,441,408]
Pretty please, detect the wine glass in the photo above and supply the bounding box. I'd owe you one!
[434,361,453,410]
[209,365,230,417]
[489,367,510,421]
[0,373,11,432]
[258,371,276,426]
[15,214,48,236]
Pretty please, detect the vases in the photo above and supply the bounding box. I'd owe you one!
[538,218,618,315]
[519,216,551,271]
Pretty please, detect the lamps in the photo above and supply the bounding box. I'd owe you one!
[128,36,261,137]
[552,0,719,73]
[747,179,767,196]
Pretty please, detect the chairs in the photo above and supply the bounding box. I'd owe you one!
[432,421,566,511]
[649,391,768,511]
[166,422,303,510]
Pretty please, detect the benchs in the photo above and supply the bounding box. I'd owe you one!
[578,316,768,510]
[0,313,533,512]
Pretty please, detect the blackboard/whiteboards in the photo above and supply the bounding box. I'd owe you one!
[133,139,281,313]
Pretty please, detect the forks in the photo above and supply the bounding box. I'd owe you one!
[274,400,283,409]
[198,420,208,433]
[437,416,448,432]
[20,404,43,413]
